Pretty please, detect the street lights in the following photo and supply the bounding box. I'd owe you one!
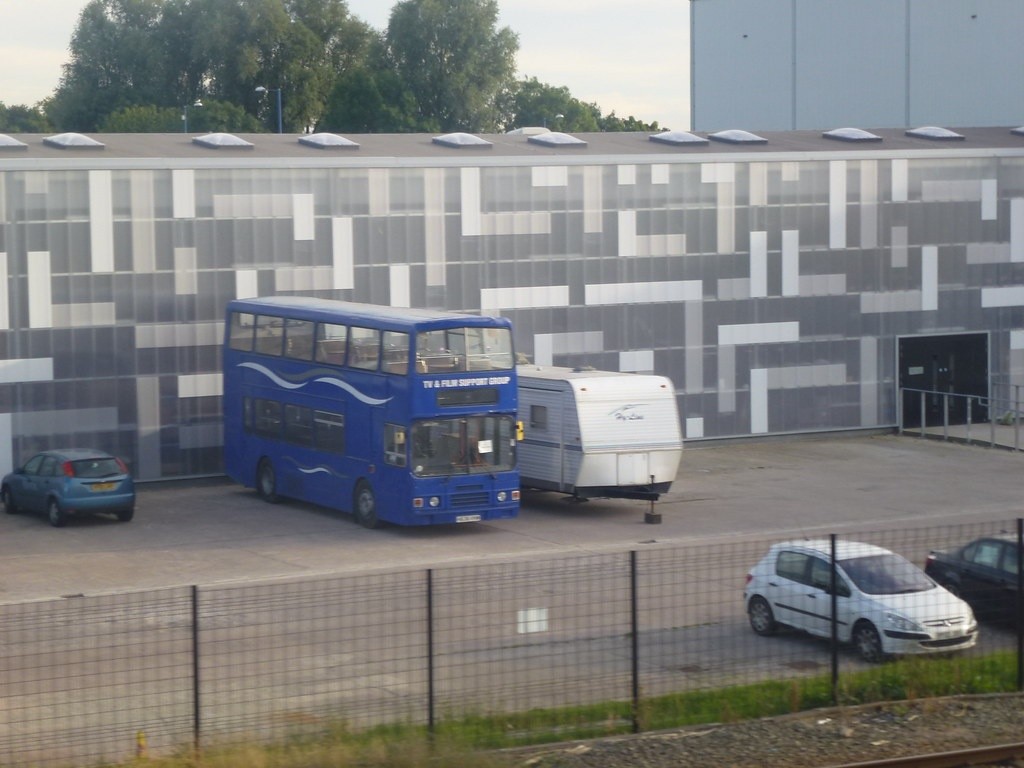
[183,100,203,133]
[542,114,564,129]
[255,86,283,134]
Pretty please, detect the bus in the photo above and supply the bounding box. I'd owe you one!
[220,293,527,531]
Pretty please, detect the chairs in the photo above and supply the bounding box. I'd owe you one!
[286,334,493,374]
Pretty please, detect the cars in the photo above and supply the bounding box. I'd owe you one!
[0,445,137,528]
[739,538,980,664]
[922,535,1019,627]
